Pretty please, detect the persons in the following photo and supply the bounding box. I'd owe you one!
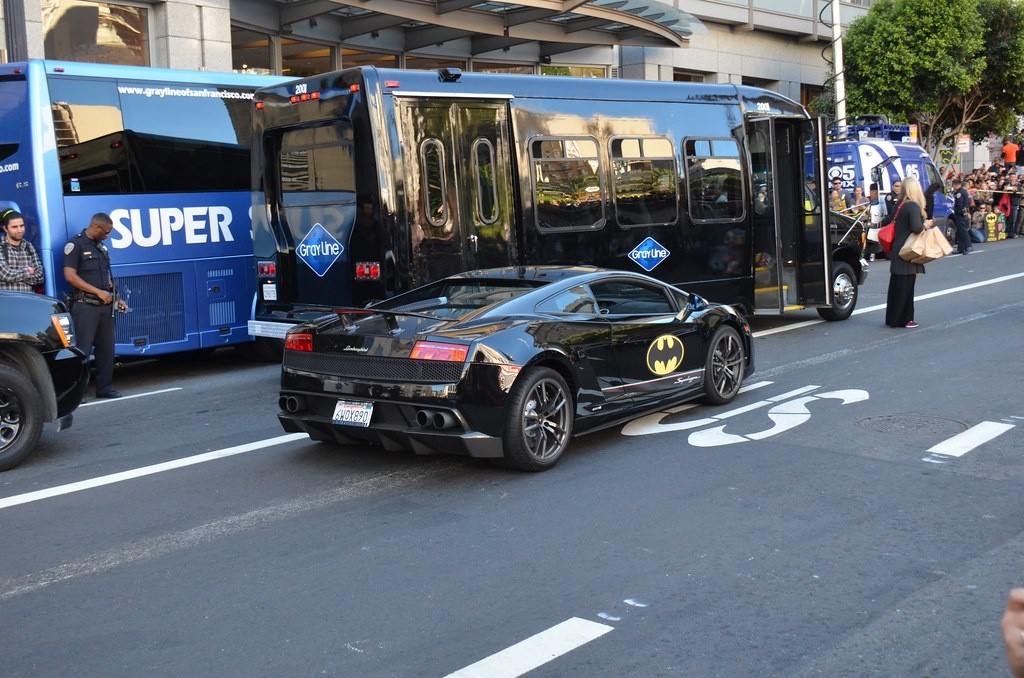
[1001,138,1021,170]
[868,183,879,205]
[0,208,44,292]
[715,175,870,267]
[939,158,1024,242]
[62,213,127,404]
[885,177,936,328]
[952,180,973,255]
[885,181,900,214]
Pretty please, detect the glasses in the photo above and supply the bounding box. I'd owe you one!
[833,182,842,185]
[980,207,985,209]
[893,184,901,188]
[95,224,111,234]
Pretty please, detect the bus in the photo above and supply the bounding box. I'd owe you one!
[244,61,880,342]
[1,54,308,368]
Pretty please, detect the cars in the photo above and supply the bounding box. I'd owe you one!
[0,288,91,473]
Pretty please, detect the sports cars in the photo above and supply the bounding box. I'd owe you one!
[276,265,758,473]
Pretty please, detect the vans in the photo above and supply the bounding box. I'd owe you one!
[802,115,958,257]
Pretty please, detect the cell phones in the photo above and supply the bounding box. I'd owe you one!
[120,307,133,312]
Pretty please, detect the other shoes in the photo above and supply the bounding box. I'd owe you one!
[952,248,962,254]
[905,320,918,328]
[870,253,875,261]
[859,258,869,267]
[962,246,973,255]
[96,389,121,398]
[1007,233,1018,238]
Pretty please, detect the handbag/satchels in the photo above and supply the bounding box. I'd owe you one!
[910,230,943,265]
[897,227,926,262]
[927,223,953,256]
[877,220,896,255]
